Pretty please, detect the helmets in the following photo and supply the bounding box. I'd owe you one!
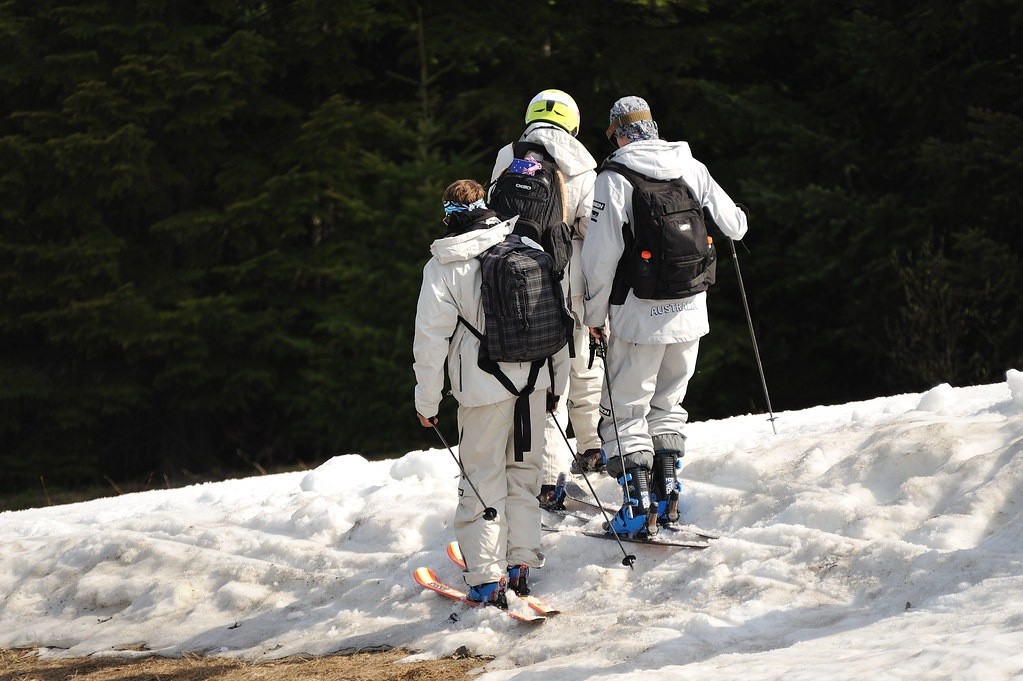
[524,88,580,139]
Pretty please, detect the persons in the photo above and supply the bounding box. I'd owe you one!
[413,180,570,610]
[580,96,748,536]
[489,88,607,509]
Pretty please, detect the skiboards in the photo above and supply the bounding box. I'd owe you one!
[415,538,563,623]
[541,481,721,550]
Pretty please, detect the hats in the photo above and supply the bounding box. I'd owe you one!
[609,96,658,139]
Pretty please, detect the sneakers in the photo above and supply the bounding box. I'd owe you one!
[466,582,499,602]
[538,485,566,513]
[506,564,521,586]
[570,449,607,473]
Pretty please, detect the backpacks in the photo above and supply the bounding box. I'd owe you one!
[457,234,575,373]
[487,142,578,280]
[601,162,716,300]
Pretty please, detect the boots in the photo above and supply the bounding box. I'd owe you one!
[648,451,678,520]
[602,466,651,535]
